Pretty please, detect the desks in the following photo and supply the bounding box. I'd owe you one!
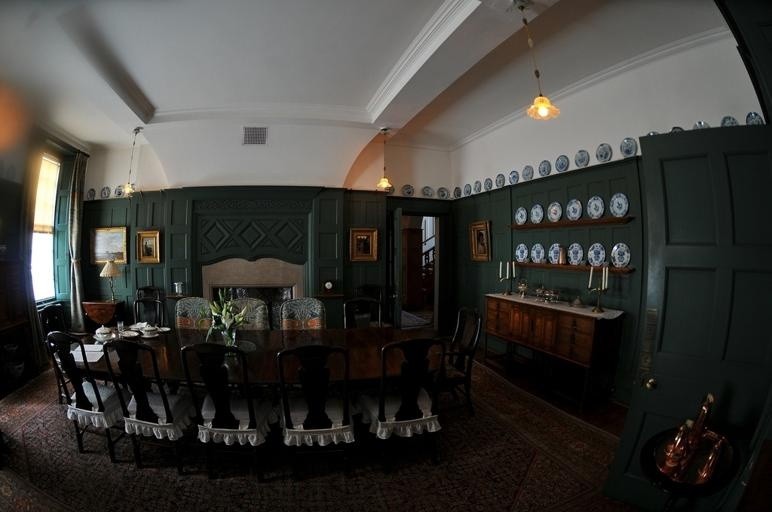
[483,290,624,369]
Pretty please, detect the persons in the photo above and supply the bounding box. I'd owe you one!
[477,232,484,253]
[145,241,150,256]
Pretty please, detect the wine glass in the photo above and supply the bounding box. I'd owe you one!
[517,278,564,303]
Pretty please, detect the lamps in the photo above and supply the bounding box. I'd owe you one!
[377,127,392,188]
[509,0,561,120]
[122,126,144,195]
[100,260,122,300]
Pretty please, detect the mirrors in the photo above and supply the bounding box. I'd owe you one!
[394,208,439,330]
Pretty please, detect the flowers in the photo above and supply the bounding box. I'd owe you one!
[206,299,252,343]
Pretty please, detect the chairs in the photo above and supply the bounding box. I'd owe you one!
[102,340,194,472]
[221,299,268,331]
[36,301,110,404]
[133,287,164,329]
[273,344,352,473]
[46,331,131,463]
[182,342,278,473]
[176,296,216,330]
[429,307,480,419]
[279,298,328,331]
[360,338,449,471]
[342,297,383,329]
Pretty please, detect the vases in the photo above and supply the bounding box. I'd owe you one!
[221,330,237,348]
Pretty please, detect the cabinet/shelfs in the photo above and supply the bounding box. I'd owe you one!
[510,215,635,275]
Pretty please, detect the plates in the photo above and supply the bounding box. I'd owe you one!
[401,184,415,197]
[473,180,481,193]
[721,116,739,126]
[91,320,172,343]
[85,184,125,199]
[692,120,711,130]
[596,143,612,162]
[511,194,633,269]
[508,171,519,185]
[437,187,450,200]
[421,186,434,198]
[620,136,637,157]
[521,165,534,181]
[453,187,461,198]
[484,177,492,191]
[538,160,551,176]
[464,183,471,196]
[574,149,590,169]
[746,113,764,125]
[648,127,684,136]
[496,174,505,188]
[555,155,569,172]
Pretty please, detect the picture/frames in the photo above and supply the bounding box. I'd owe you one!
[135,230,160,264]
[349,227,377,263]
[468,220,491,262]
[89,226,127,266]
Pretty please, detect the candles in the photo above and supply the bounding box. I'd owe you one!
[602,266,608,290]
[588,266,593,288]
[499,261,502,278]
[506,262,509,278]
[512,261,515,277]
